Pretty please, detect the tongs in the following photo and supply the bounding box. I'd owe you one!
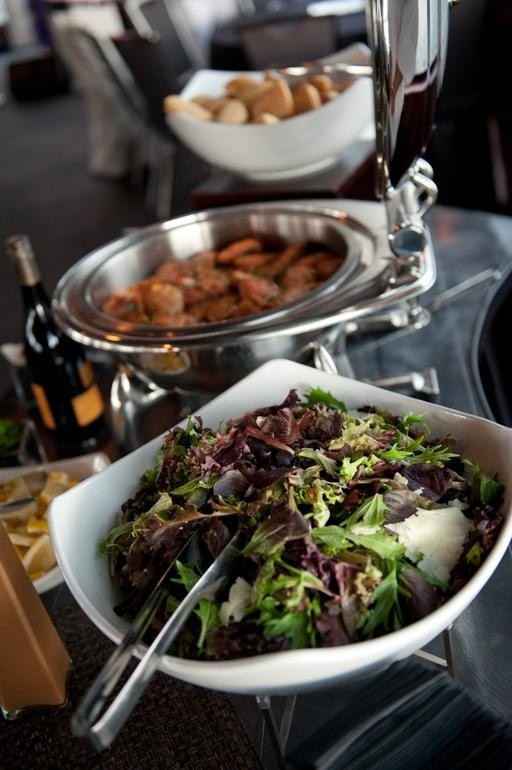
[68,478,251,754]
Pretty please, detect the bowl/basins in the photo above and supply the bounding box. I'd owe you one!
[40,356,512,708]
[163,68,374,184]
[48,200,435,395]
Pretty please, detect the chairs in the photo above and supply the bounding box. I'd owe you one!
[50,8,367,221]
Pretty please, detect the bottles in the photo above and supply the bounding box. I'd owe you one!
[4,229,116,462]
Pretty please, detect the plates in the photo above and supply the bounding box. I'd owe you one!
[3,451,116,605]
